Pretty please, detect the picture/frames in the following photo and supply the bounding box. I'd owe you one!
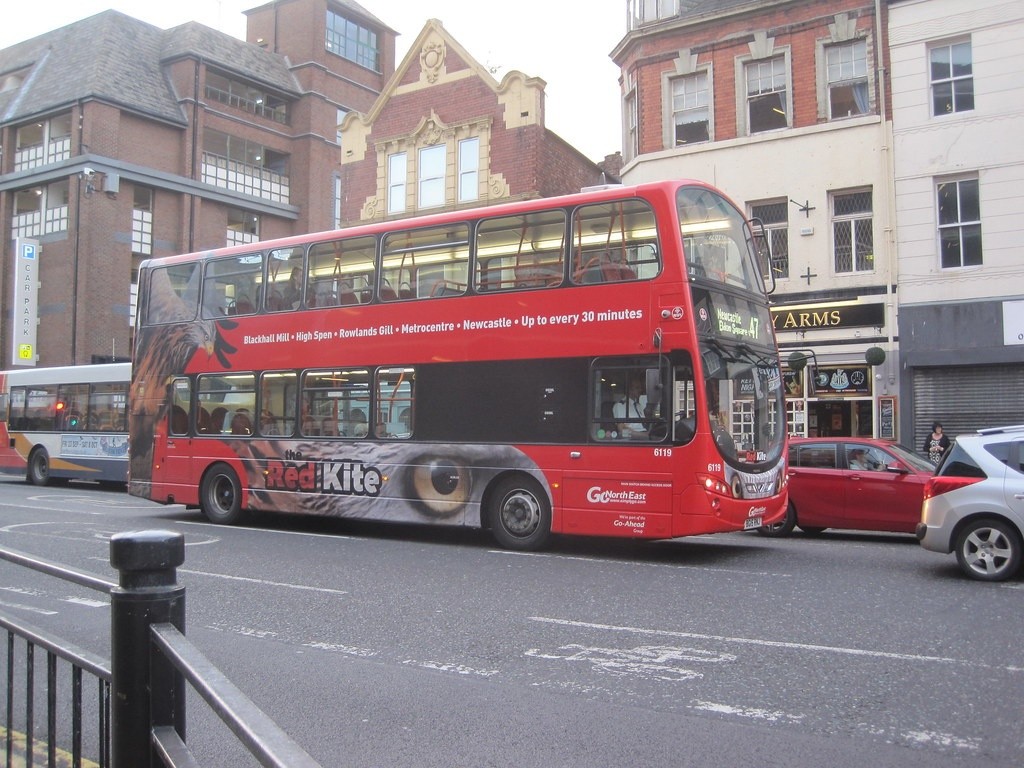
[878,396,897,439]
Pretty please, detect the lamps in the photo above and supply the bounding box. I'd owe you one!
[256,94,263,105]
[253,151,260,160]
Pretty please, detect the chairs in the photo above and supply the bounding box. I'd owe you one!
[17,409,123,434]
[214,256,635,313]
[174,403,412,442]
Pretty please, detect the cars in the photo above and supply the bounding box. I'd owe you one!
[757,436,936,539]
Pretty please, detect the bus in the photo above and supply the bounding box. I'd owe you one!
[137,180,828,555]
[0,362,333,489]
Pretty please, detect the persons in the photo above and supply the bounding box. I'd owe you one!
[282,267,311,310]
[849,449,884,472]
[354,424,367,437]
[613,374,656,439]
[923,423,950,466]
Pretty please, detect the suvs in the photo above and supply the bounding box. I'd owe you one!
[916,426,1024,583]
